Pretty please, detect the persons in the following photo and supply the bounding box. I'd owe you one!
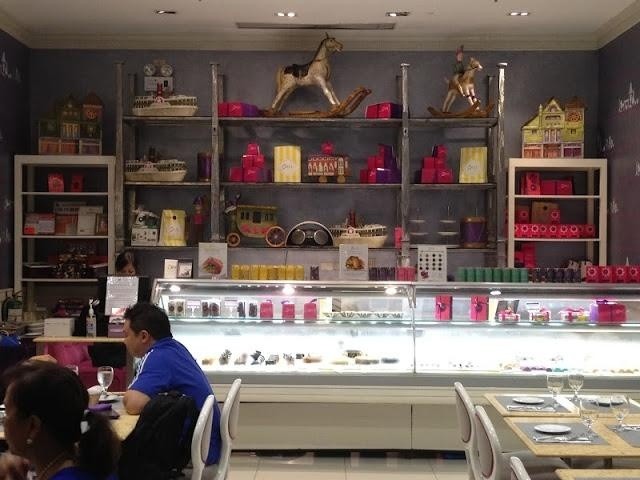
[119,301,222,469]
[0,362,120,479]
[114,251,141,277]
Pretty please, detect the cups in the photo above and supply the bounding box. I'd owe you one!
[231,265,305,280]
[456,268,529,283]
[531,268,582,283]
[311,265,320,280]
[369,267,396,280]
[397,267,416,281]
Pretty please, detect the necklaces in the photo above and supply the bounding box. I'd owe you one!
[35,448,72,479]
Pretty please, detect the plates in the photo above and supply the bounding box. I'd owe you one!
[26,322,44,335]
[437,231,459,236]
[512,396,545,403]
[440,220,456,223]
[594,399,623,406]
[534,423,571,434]
[446,244,460,249]
[409,233,429,236]
[95,395,120,401]
[499,321,519,325]
[561,321,591,325]
[408,219,424,223]
[529,321,550,325]
[409,244,416,248]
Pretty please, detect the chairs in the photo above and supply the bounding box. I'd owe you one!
[445,377,529,478]
[187,376,241,479]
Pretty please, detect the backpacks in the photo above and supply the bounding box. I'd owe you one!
[117,390,197,480]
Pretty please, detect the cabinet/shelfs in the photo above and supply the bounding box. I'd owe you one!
[14,153,119,313]
[507,154,610,267]
[112,56,510,278]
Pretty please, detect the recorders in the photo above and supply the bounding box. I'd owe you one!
[285,221,335,247]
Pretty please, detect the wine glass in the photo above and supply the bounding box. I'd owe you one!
[97,366,114,398]
[568,372,585,402]
[609,396,630,432]
[579,400,600,438]
[547,373,564,407]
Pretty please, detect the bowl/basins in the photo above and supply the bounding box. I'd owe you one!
[322,311,404,319]
